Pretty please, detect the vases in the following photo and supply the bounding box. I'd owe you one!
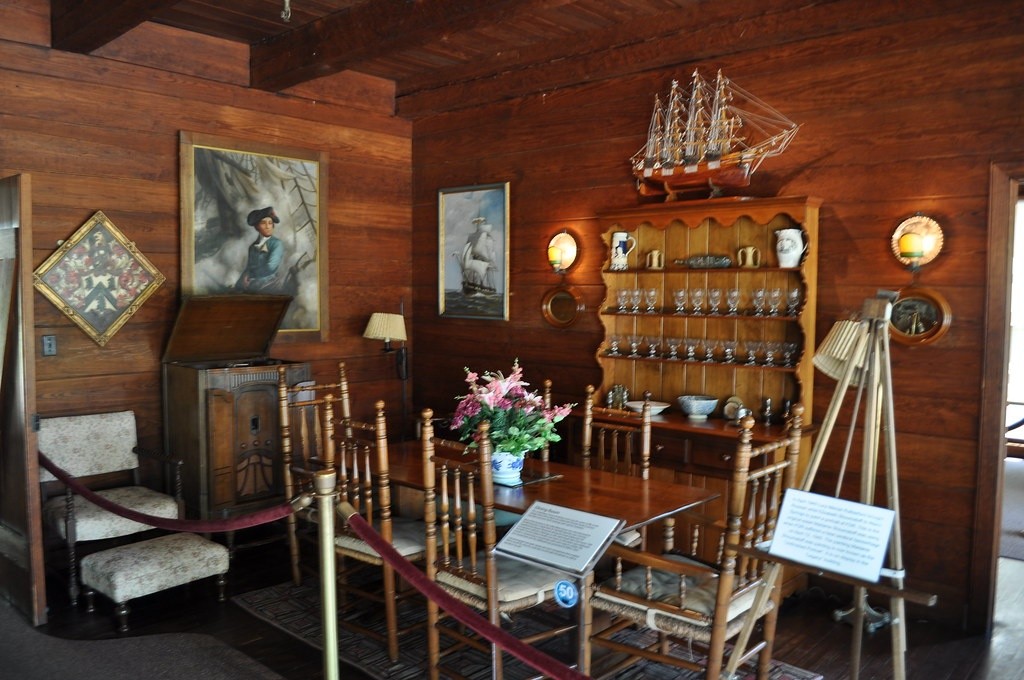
[490,449,530,484]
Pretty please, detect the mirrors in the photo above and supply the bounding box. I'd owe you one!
[890,215,944,265]
[547,231,579,274]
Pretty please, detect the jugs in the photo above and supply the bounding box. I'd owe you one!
[775,229,808,268]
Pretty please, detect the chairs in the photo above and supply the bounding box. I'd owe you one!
[421,406,586,680]
[36,408,184,615]
[580,402,806,680]
[582,384,652,626]
[420,379,552,551]
[323,391,466,665]
[277,363,401,603]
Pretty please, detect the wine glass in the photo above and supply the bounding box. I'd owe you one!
[614,288,801,317]
[607,334,798,369]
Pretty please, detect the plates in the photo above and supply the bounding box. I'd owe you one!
[723,396,743,419]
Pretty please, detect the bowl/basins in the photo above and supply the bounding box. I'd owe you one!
[678,396,719,420]
[626,401,671,415]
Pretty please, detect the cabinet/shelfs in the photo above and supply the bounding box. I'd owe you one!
[161,294,312,538]
[590,194,822,603]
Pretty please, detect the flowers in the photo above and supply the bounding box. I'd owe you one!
[448,356,578,459]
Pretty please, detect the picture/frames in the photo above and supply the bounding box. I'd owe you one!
[179,129,330,344]
[33,211,166,348]
[437,181,511,322]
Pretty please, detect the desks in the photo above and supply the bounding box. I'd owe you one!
[308,437,723,680]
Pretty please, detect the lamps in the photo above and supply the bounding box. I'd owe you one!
[363,311,408,352]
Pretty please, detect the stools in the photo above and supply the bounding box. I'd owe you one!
[79,532,229,635]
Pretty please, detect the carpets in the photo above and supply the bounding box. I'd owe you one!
[229,561,824,680]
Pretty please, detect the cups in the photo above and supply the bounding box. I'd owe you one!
[610,232,636,270]
